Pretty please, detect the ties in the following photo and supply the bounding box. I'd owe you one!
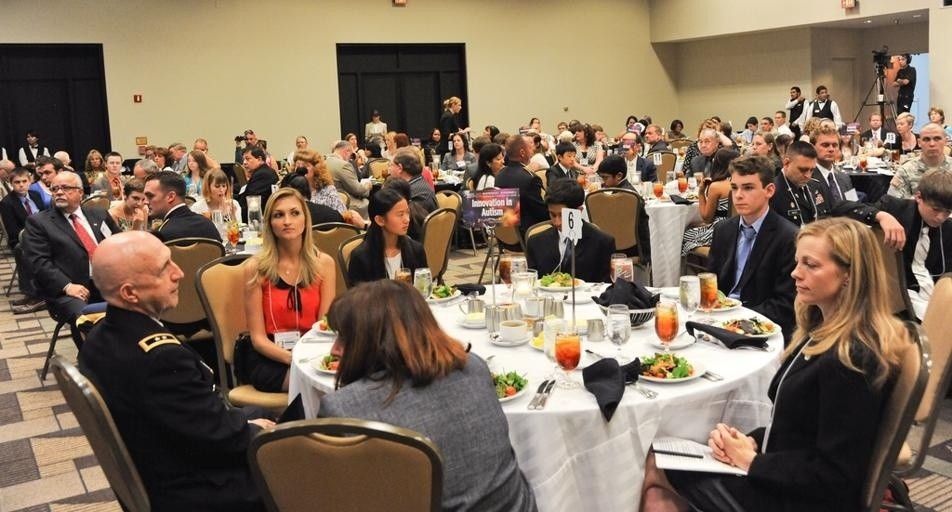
[69,214,97,264]
[907,227,934,321]
[25,199,33,217]
[827,172,842,204]
[727,226,757,298]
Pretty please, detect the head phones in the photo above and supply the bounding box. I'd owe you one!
[907,54,911,63]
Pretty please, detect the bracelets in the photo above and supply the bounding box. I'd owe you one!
[897,79,901,82]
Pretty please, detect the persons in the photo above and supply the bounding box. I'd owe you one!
[316,279,538,511]
[74,229,270,512]
[893,54,916,114]
[0,86,952,394]
[642,218,911,512]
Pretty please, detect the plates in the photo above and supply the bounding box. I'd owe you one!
[697,297,741,313]
[497,379,531,402]
[458,316,487,328]
[712,321,783,341]
[419,285,462,304]
[312,355,339,375]
[489,330,529,347]
[634,357,706,384]
[559,291,596,305]
[488,329,532,347]
[539,277,584,291]
[309,320,337,334]
[653,334,695,349]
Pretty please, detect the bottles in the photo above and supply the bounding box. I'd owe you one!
[526,297,544,313]
[511,269,537,295]
[501,253,514,285]
[699,273,718,303]
[395,267,412,286]
[460,298,485,322]
[415,269,432,297]
[680,276,701,311]
[512,256,528,281]
[587,319,603,341]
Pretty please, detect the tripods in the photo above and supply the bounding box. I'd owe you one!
[853,75,898,130]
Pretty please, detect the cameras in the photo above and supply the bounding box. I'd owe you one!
[234,136,247,142]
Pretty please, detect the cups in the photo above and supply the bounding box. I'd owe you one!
[849,148,900,173]
[584,170,705,205]
[428,155,443,183]
[500,320,527,341]
[202,196,263,254]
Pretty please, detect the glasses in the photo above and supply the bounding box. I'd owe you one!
[50,185,81,192]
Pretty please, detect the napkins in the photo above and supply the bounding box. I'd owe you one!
[581,357,640,421]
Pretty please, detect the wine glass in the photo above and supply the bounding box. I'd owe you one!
[609,253,633,287]
[656,302,680,356]
[546,321,568,383]
[607,304,631,365]
[554,327,582,389]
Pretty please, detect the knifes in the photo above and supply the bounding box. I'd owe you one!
[537,379,555,412]
[528,379,549,410]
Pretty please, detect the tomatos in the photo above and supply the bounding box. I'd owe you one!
[332,359,340,369]
[656,373,664,378]
[505,386,516,395]
[320,322,327,329]
[766,322,773,328]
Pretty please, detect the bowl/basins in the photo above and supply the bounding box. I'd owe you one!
[598,302,656,331]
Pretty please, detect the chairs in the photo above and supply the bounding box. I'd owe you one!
[3,139,895,397]
[247,416,445,511]
[48,355,150,512]
[863,320,933,512]
[887,272,952,477]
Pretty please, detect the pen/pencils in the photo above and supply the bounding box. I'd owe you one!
[651,449,703,458]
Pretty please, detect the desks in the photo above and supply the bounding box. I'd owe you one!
[290,281,784,418]
